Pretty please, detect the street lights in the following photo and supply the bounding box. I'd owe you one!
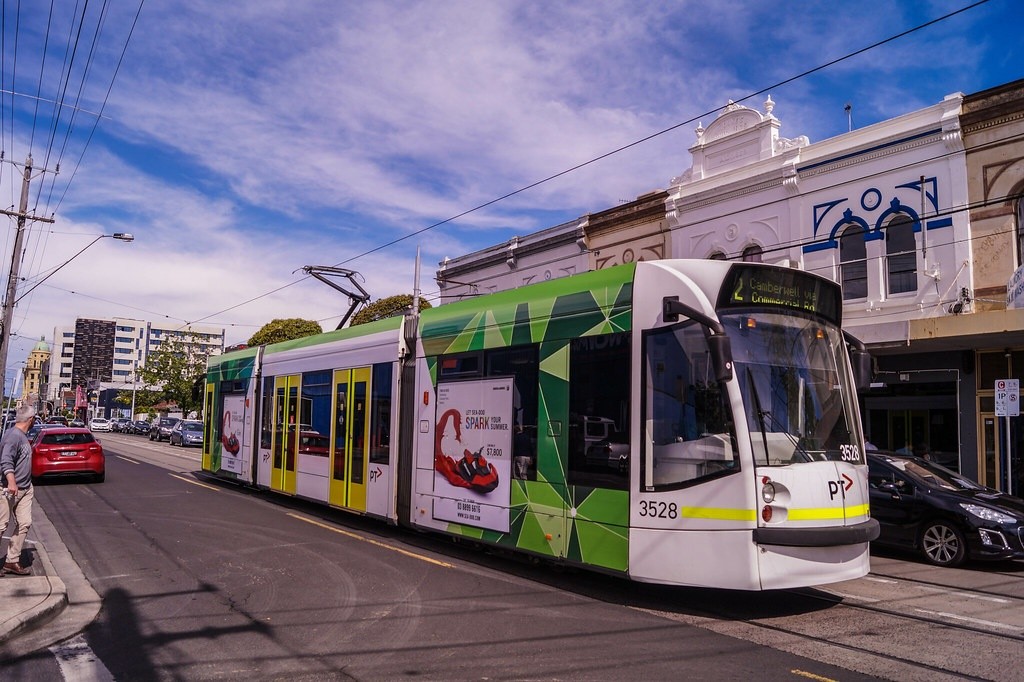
[0,233,135,414]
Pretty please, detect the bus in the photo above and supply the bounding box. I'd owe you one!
[200,257,881,591]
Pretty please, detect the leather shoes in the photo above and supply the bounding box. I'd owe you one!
[0,570,4,577]
[2,562,30,575]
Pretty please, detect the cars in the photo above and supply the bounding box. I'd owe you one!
[3,408,70,444]
[149,416,180,442]
[31,427,105,486]
[169,419,204,448]
[826,451,1024,568]
[88,418,110,433]
[109,418,151,436]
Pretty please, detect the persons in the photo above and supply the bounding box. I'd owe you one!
[0,405,37,577]
[864,434,878,450]
[896,442,913,455]
